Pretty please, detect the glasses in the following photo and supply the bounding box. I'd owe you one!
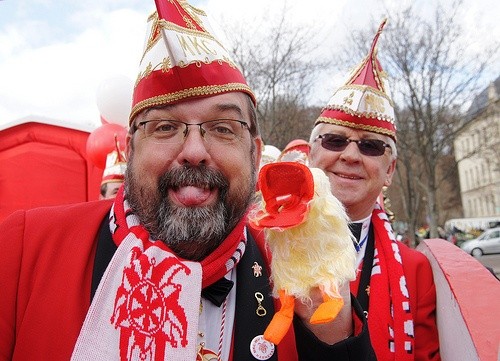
[312,133,392,157]
[131,119,256,144]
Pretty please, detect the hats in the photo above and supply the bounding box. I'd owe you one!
[313,18,397,143]
[129,0,256,127]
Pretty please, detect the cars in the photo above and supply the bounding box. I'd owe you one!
[461,228,500,257]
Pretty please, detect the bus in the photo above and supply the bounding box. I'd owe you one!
[443,217,500,239]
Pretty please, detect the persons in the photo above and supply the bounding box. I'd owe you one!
[391,221,486,249]
[1,66,377,361]
[307,92,441,361]
[98,169,129,200]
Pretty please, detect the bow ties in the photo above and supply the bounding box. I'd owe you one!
[346,223,363,247]
[200,277,234,308]
